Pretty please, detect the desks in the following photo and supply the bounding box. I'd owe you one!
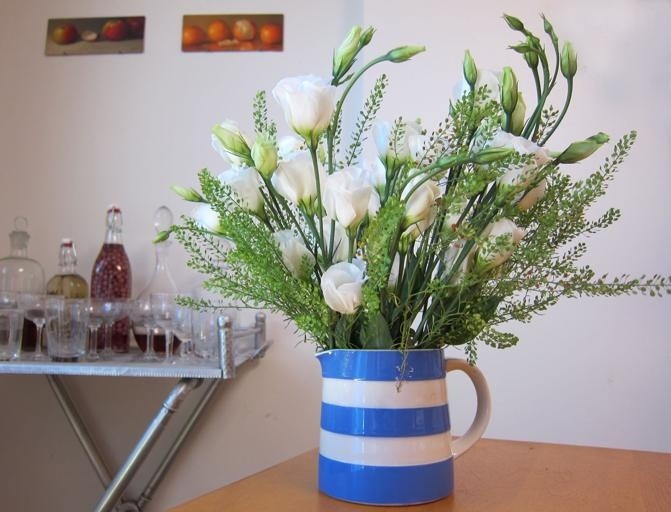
[172,436,670,510]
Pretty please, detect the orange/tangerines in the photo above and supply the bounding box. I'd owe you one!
[260,24,283,45]
[232,20,256,40]
[183,25,206,47]
[207,20,232,41]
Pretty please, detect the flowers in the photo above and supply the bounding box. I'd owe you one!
[151,12,671,391]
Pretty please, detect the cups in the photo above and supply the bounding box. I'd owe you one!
[314,348,491,508]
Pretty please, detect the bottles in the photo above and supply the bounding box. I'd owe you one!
[0,214,46,354]
[47,232,90,361]
[91,207,132,354]
[134,204,185,358]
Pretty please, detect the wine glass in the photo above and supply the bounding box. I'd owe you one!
[0,292,236,366]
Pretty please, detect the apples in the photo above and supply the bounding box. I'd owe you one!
[102,19,129,40]
[53,23,76,44]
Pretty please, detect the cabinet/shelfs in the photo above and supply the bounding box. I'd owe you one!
[0,314,272,510]
